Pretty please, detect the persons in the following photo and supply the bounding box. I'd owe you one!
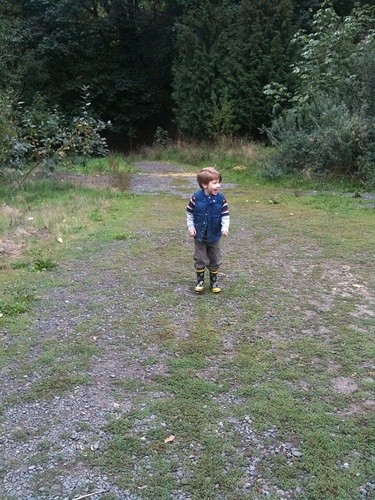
[186,167,229,293]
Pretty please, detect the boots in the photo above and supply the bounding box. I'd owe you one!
[209,271,222,293]
[194,269,206,292]
